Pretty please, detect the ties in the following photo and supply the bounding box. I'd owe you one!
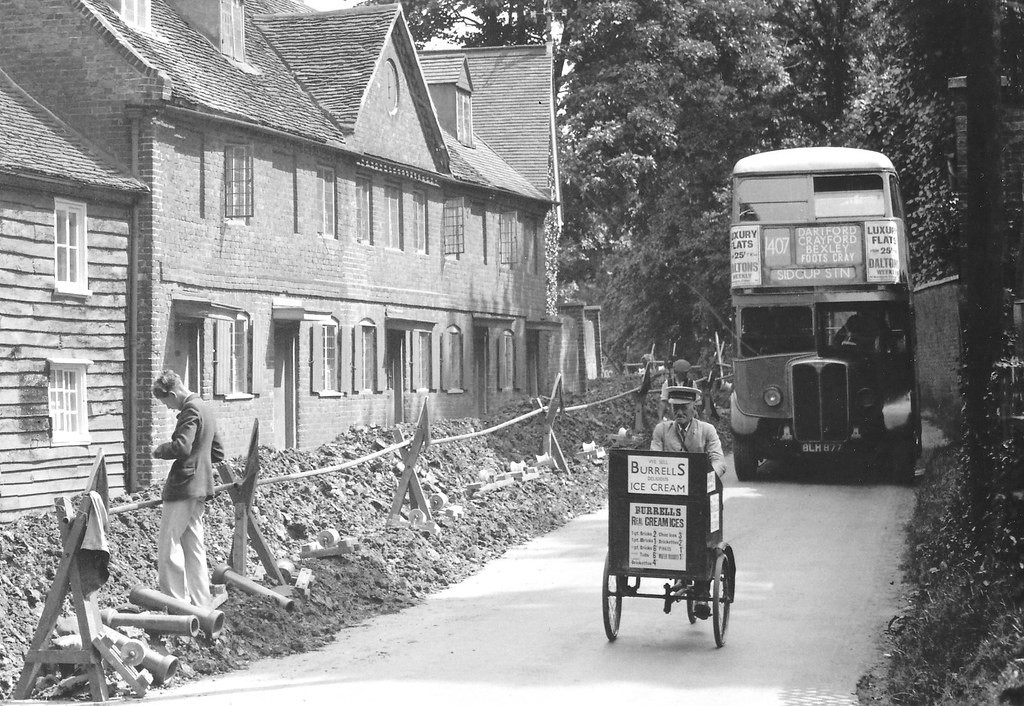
[681,428,686,442]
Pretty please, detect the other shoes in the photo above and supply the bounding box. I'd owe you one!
[694,601,710,619]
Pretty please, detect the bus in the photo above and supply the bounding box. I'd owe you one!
[730,151,922,484]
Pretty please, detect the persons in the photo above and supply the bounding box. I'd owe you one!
[153,369,225,609]
[649,385,728,620]
[657,359,698,421]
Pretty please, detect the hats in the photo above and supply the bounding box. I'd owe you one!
[673,360,691,372]
[665,386,702,404]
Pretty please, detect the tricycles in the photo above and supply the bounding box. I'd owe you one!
[602,448,736,647]
